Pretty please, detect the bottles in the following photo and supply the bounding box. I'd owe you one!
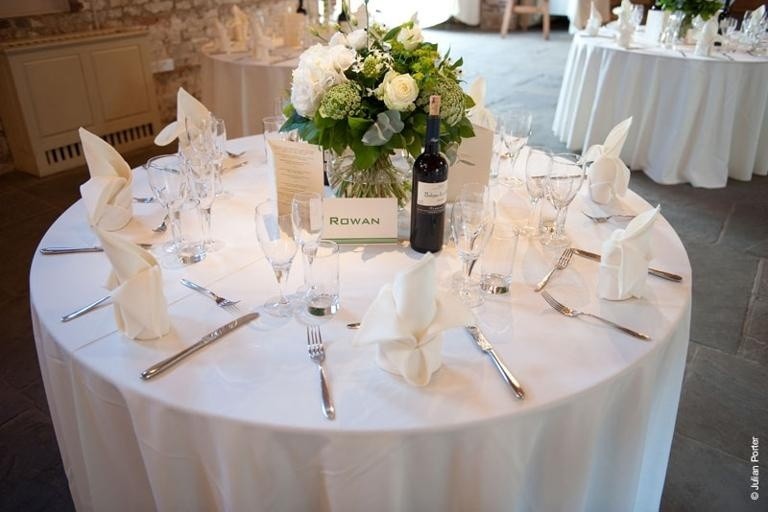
[411,94,448,257]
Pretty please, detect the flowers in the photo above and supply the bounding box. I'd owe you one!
[278,0,475,203]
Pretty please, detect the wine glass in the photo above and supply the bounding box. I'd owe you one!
[518,147,586,243]
[500,106,532,185]
[450,180,495,304]
[624,7,766,56]
[256,193,326,315]
[145,116,227,264]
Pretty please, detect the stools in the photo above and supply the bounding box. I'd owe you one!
[499,0,551,42]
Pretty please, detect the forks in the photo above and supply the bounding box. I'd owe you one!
[539,290,652,347]
[303,324,339,421]
[536,246,574,295]
[583,212,639,223]
[178,276,240,310]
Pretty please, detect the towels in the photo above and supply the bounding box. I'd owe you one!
[208,4,272,59]
[596,206,661,301]
[584,1,602,35]
[696,9,724,56]
[77,86,213,340]
[358,250,478,387]
[579,115,633,205]
[611,0,636,46]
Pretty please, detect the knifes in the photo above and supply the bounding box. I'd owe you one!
[574,247,683,285]
[465,325,524,399]
[140,311,263,383]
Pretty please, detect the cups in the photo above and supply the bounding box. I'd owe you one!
[262,115,289,168]
[300,239,346,317]
[483,220,517,295]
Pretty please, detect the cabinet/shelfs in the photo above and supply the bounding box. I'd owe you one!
[0,24,165,178]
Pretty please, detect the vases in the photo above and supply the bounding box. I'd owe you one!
[325,148,416,211]
